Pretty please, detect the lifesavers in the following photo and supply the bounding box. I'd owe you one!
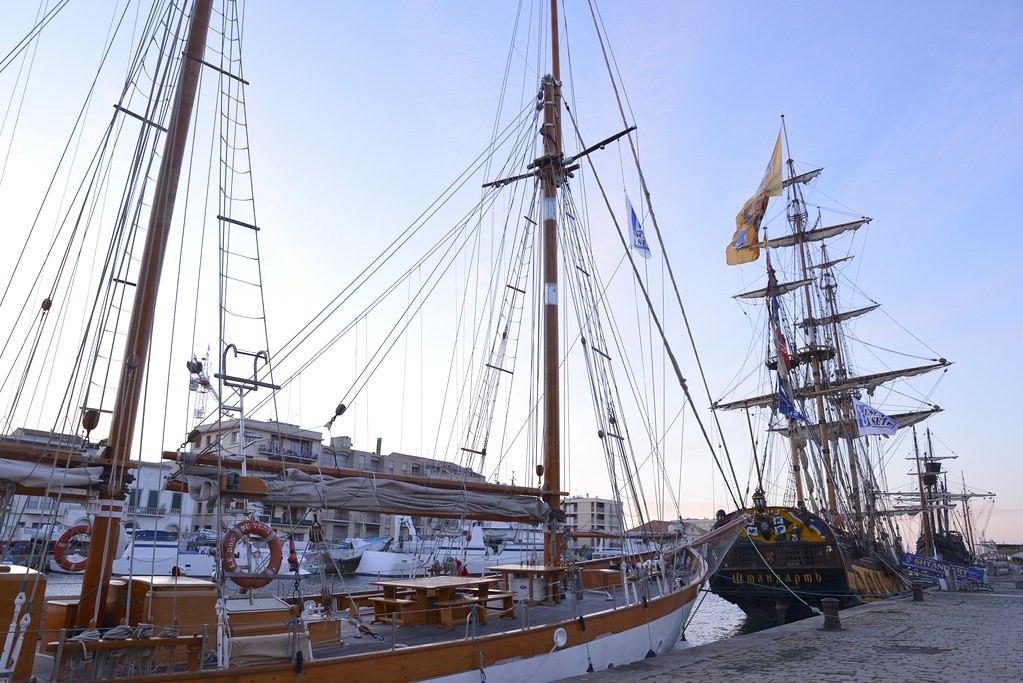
[52,524,94,572]
[220,519,284,590]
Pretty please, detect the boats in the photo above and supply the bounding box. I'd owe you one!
[237,515,662,579]
[50,529,223,577]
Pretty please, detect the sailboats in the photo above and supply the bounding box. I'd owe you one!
[0,1,761,683]
[701,110,1000,623]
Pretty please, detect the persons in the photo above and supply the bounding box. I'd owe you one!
[446,556,469,576]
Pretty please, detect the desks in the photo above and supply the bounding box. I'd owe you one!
[367,575,504,625]
[584,567,621,591]
[486,564,568,606]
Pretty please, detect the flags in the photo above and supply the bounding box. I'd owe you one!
[778,372,813,423]
[726,121,783,265]
[625,193,651,259]
[772,281,791,372]
[852,395,899,436]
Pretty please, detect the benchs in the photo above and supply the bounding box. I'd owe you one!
[345,588,517,631]
